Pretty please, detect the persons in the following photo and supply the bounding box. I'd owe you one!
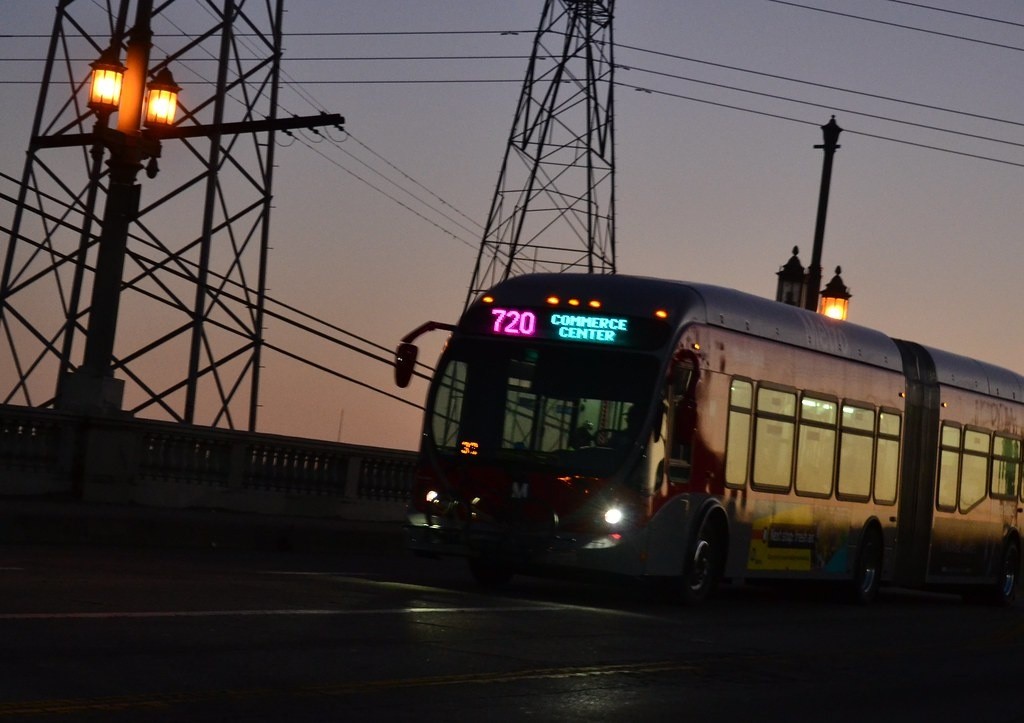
[605,405,637,446]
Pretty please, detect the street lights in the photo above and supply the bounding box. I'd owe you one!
[57,0,182,414]
[776,114,853,321]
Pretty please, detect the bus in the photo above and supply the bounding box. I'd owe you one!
[394,274,1024,607]
